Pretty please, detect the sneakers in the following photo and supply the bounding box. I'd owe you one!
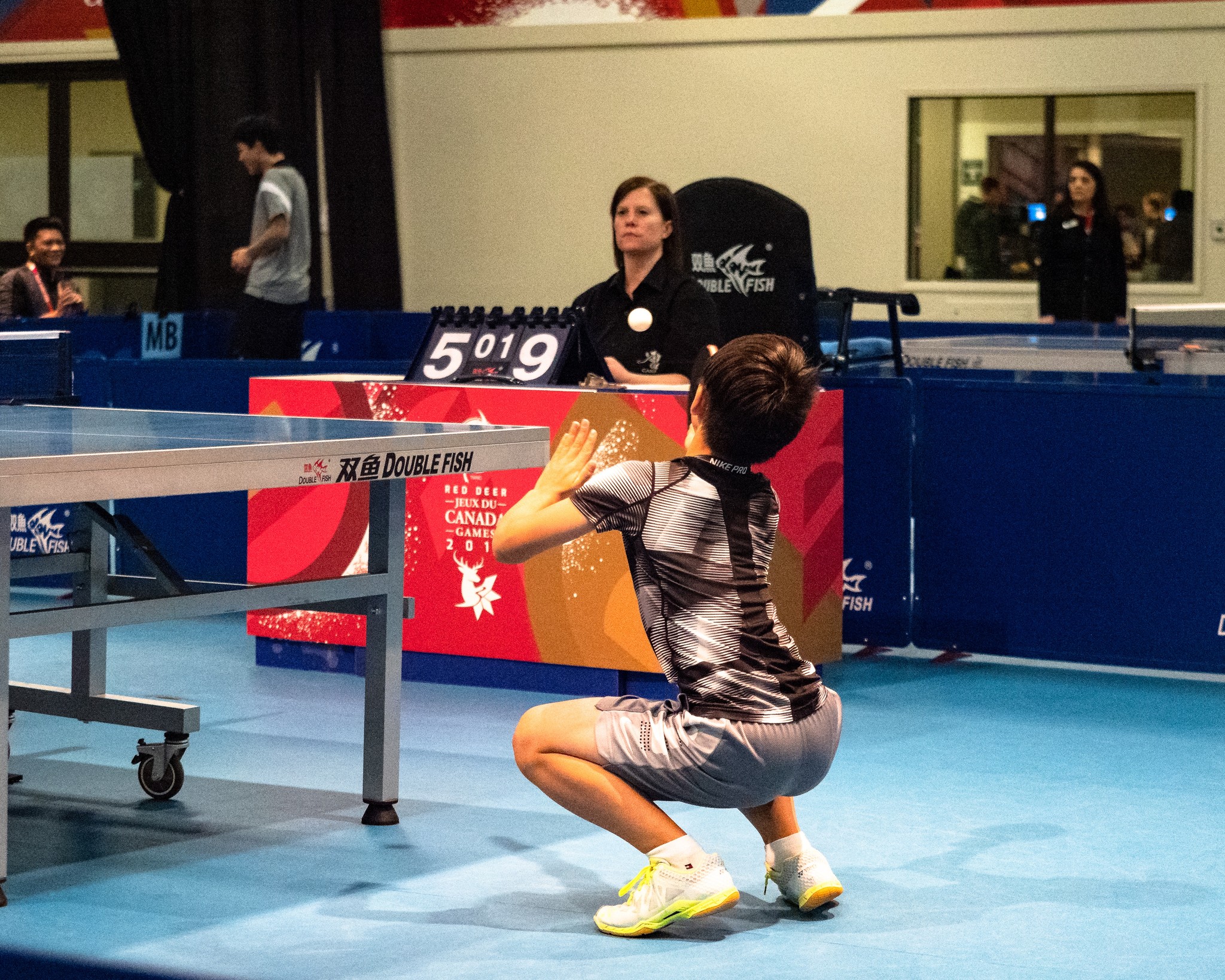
[594,852,741,935]
[763,854,844,913]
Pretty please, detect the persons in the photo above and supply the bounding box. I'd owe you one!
[955,176,1027,283]
[1153,190,1194,282]
[231,114,311,360]
[0,216,84,324]
[571,177,721,385]
[493,334,842,936]
[1036,160,1127,324]
[1114,205,1145,271]
[1143,192,1170,281]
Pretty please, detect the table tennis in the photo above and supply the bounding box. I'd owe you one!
[627,308,653,333]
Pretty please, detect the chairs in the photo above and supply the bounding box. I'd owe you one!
[674,177,921,378]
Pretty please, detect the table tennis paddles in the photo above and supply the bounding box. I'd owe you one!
[686,344,719,430]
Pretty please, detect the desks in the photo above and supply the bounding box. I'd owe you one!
[247,374,843,700]
[0,399,551,825]
